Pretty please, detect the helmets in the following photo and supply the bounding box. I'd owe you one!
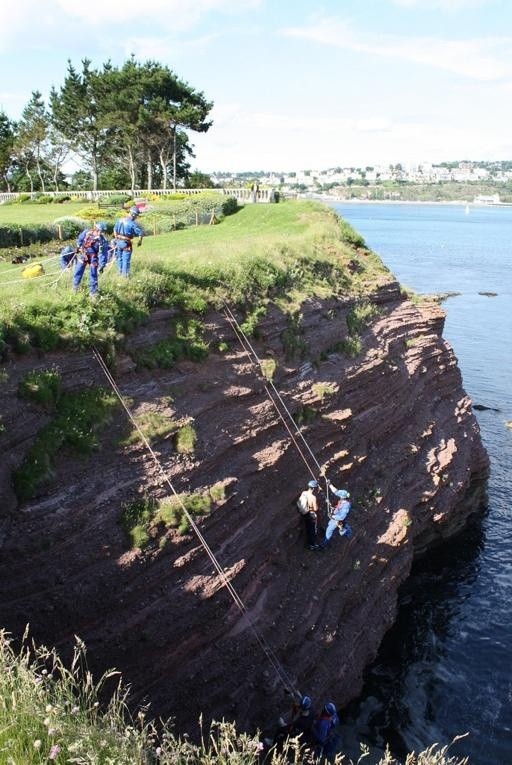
[337,489,348,496]
[300,696,312,709]
[325,702,336,716]
[308,480,320,487]
[130,207,139,214]
[97,222,108,231]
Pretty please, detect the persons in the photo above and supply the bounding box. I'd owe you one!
[71,221,108,295]
[113,206,144,276]
[314,479,352,550]
[251,181,259,202]
[296,480,319,550]
[264,689,340,751]
